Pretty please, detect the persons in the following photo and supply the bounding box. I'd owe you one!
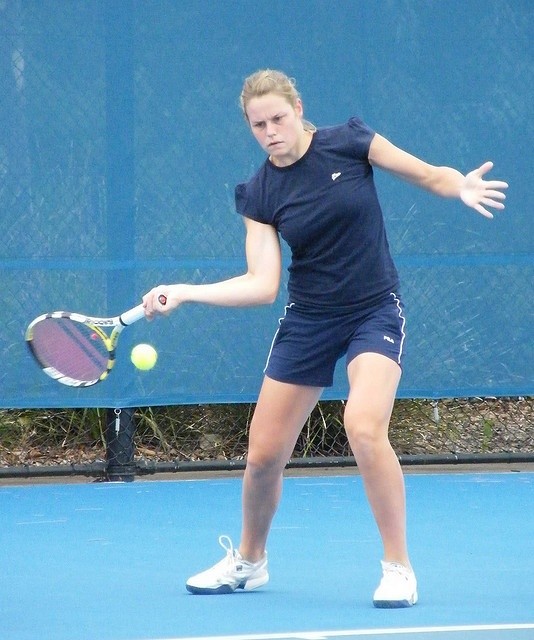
[142,68,509,609]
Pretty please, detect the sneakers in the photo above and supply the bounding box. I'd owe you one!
[186,535,268,596]
[373,561,417,608]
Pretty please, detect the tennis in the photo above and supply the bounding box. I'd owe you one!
[131,344,157,369]
